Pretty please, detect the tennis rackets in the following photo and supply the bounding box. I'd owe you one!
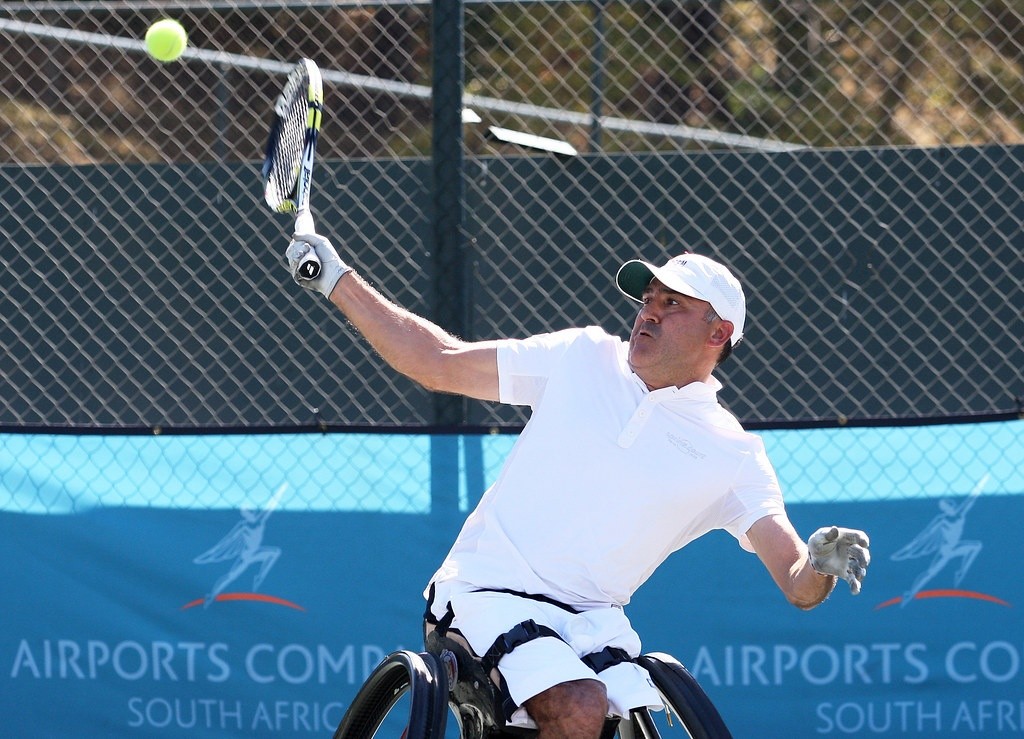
[261,56,322,280]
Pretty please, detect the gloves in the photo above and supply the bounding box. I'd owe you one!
[809,527,870,595]
[286,233,353,301]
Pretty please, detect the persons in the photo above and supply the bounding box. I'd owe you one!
[285,230,871,739]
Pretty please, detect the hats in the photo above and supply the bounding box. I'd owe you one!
[616,252,747,348]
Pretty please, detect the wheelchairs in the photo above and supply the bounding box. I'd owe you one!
[329,650,730,739]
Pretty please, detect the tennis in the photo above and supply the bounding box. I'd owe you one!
[145,18,187,63]
